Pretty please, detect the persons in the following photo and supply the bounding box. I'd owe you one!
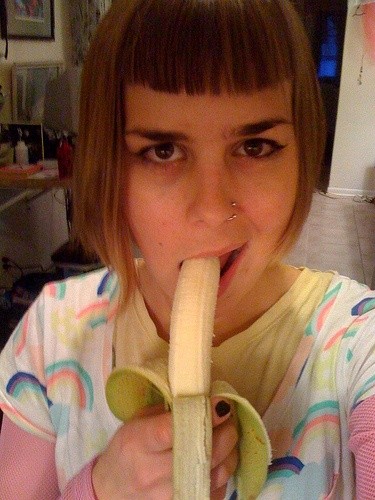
[0,0,375,500]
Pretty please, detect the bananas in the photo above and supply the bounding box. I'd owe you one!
[104,256,271,499]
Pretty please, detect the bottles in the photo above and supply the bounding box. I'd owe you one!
[55,130,75,182]
[14,141,29,166]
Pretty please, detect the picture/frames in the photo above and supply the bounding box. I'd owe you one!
[11,60,66,122]
[0,0,56,42]
